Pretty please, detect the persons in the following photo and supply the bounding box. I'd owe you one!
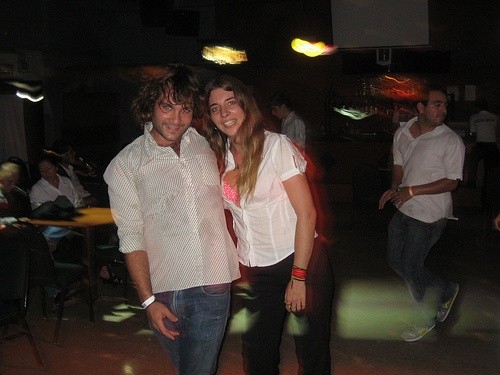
[269,89,306,158]
[466,100,500,189]
[493,213,500,231]
[196,74,333,375]
[101,63,242,375]
[377,83,466,342]
[0,157,83,218]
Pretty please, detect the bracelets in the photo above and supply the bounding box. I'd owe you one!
[408,187,414,197]
[141,295,156,309]
[289,266,310,289]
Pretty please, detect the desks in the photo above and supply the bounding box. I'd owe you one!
[18,207,115,322]
[309,141,391,203]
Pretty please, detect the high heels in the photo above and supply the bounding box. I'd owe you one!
[100,273,124,285]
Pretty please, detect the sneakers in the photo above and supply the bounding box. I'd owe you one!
[401,319,436,342]
[437,282,460,322]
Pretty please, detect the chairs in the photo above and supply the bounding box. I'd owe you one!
[7,220,93,344]
[0,222,47,369]
